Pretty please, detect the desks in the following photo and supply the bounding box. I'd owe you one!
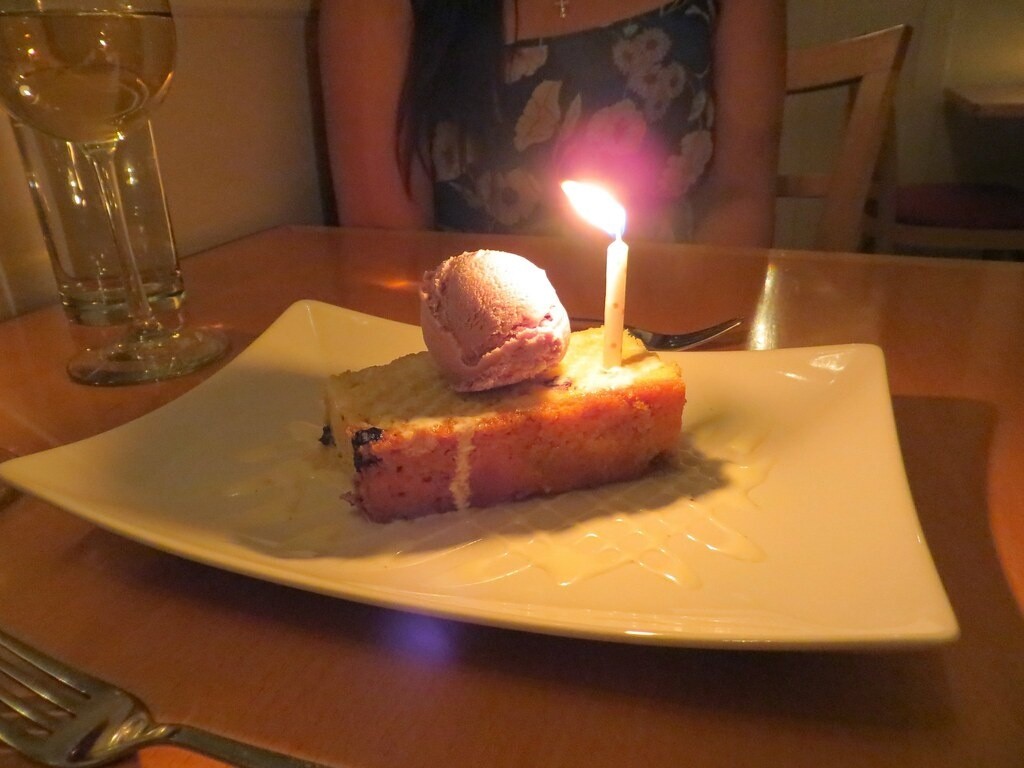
[1,223,1023,768]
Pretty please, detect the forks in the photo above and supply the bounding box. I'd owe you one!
[568,310,741,353]
[0,630,331,768]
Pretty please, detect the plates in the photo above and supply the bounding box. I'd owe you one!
[0,298,962,650]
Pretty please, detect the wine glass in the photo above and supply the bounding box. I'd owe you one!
[0,0,228,386]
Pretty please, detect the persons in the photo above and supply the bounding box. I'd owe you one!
[317,0,788,249]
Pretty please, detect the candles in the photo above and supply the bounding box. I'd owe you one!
[603,236,630,370]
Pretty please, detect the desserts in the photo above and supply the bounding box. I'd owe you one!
[321,250,688,524]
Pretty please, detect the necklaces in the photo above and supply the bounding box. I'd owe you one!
[552,0,570,19]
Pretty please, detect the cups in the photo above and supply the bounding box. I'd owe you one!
[9,112,188,327]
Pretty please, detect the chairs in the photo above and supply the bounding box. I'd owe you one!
[846,23,1024,254]
[775,22,915,249]
